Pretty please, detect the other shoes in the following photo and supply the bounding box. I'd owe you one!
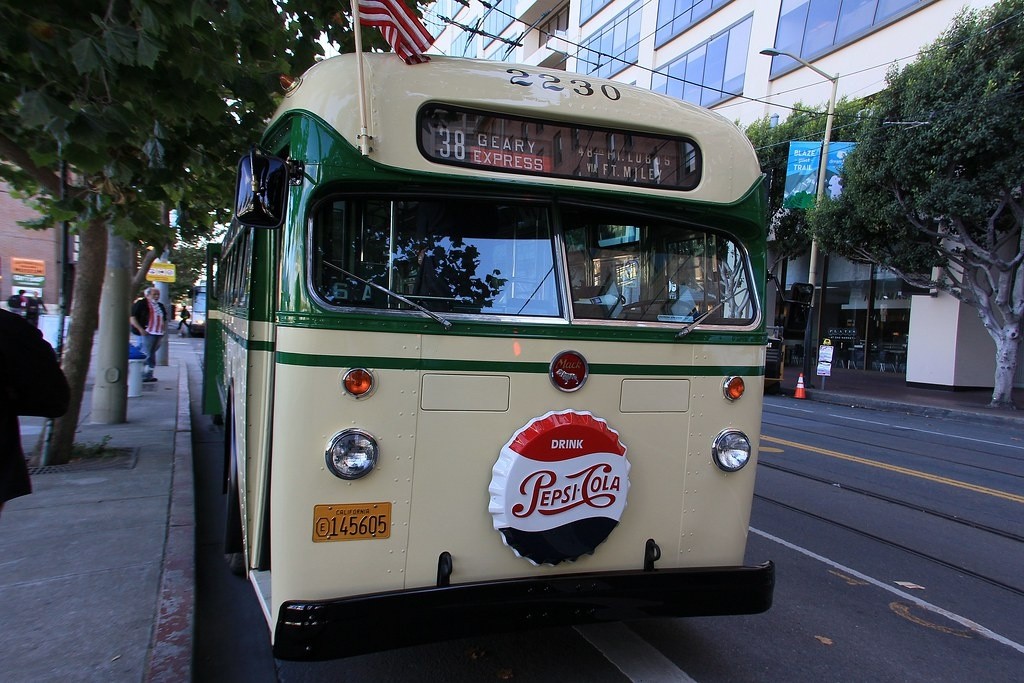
[142,378,158,382]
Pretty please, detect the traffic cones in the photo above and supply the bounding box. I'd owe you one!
[792,373,809,400]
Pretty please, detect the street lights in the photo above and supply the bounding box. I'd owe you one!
[759,51,841,389]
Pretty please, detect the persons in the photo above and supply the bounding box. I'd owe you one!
[177,305,191,330]
[0,308,70,512]
[130,287,166,383]
[8,290,48,329]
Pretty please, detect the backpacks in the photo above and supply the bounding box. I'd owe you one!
[9,296,22,309]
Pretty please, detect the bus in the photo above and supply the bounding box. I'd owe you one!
[202,50,812,659]
[187,285,206,337]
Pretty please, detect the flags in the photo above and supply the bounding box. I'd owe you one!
[358,0,436,65]
